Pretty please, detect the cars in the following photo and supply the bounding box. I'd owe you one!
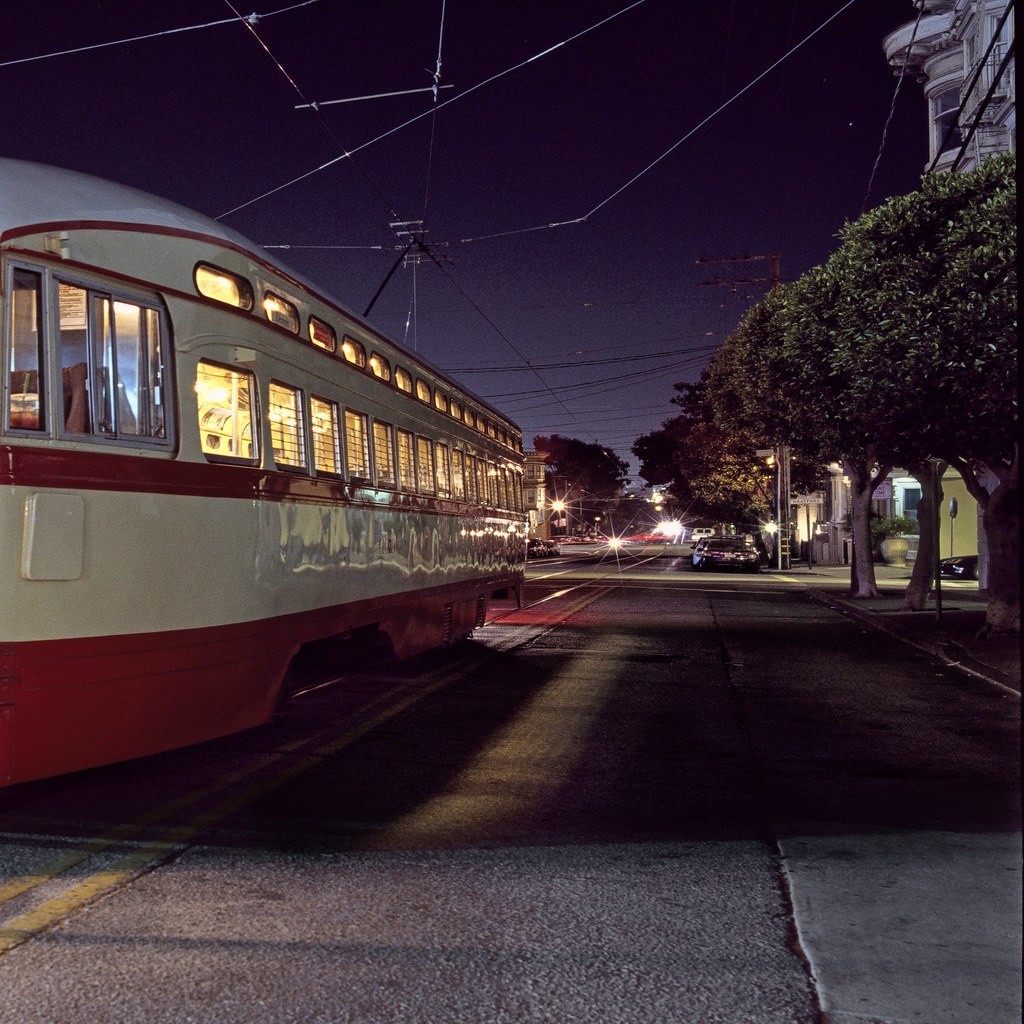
[689,527,749,566]
[526,538,561,558]
[696,538,760,574]
[940,555,980,579]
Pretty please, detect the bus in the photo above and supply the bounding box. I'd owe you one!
[1,158,526,788]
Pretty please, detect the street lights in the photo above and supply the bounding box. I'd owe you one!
[766,455,783,571]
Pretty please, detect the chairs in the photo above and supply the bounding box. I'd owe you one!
[11,362,89,434]
